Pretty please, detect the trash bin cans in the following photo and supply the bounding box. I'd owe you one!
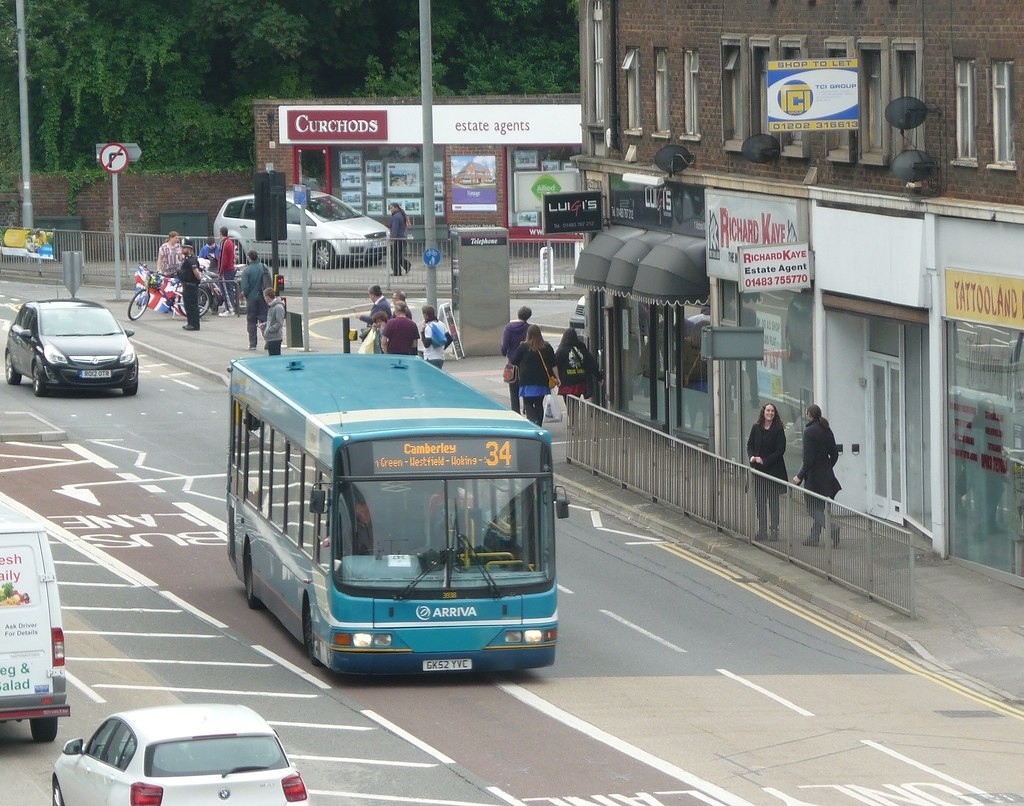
[570,314,585,343]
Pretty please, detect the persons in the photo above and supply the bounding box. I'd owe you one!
[420,305,454,370]
[511,324,561,428]
[258,288,286,355]
[554,328,603,434]
[388,203,412,276]
[356,284,420,356]
[157,227,236,330]
[746,402,789,542]
[427,480,478,520]
[320,502,387,556]
[638,301,761,434]
[32,230,50,254]
[241,250,272,350]
[794,405,842,548]
[957,398,1010,542]
[501,306,533,415]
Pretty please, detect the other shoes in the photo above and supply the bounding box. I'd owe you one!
[406,262,411,273]
[755,532,768,541]
[833,527,839,548]
[769,530,778,541]
[182,324,200,330]
[218,311,236,316]
[803,539,819,546]
[389,272,402,276]
[248,345,257,350]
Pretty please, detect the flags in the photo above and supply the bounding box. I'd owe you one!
[134,264,183,316]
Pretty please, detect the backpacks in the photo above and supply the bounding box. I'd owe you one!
[261,263,272,292]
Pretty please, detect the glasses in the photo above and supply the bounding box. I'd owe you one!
[392,297,398,300]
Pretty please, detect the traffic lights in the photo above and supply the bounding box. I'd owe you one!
[272,276,284,291]
[279,297,286,320]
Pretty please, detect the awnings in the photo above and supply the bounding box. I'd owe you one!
[573,224,710,307]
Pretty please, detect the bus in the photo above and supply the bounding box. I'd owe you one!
[226,353,570,675]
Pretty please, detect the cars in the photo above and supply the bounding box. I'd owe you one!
[51,703,309,806]
[568,295,586,342]
[4,299,139,396]
[213,191,391,268]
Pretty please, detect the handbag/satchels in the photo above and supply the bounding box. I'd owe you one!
[427,323,447,348]
[684,374,689,386]
[542,388,563,422]
[359,328,376,354]
[503,364,521,383]
[584,371,593,399]
[549,376,557,388]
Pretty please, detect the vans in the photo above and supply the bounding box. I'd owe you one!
[0,500,71,741]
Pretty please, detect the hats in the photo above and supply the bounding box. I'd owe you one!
[206,236,216,246]
[180,238,195,249]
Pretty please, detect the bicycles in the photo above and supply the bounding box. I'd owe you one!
[127,262,248,321]
